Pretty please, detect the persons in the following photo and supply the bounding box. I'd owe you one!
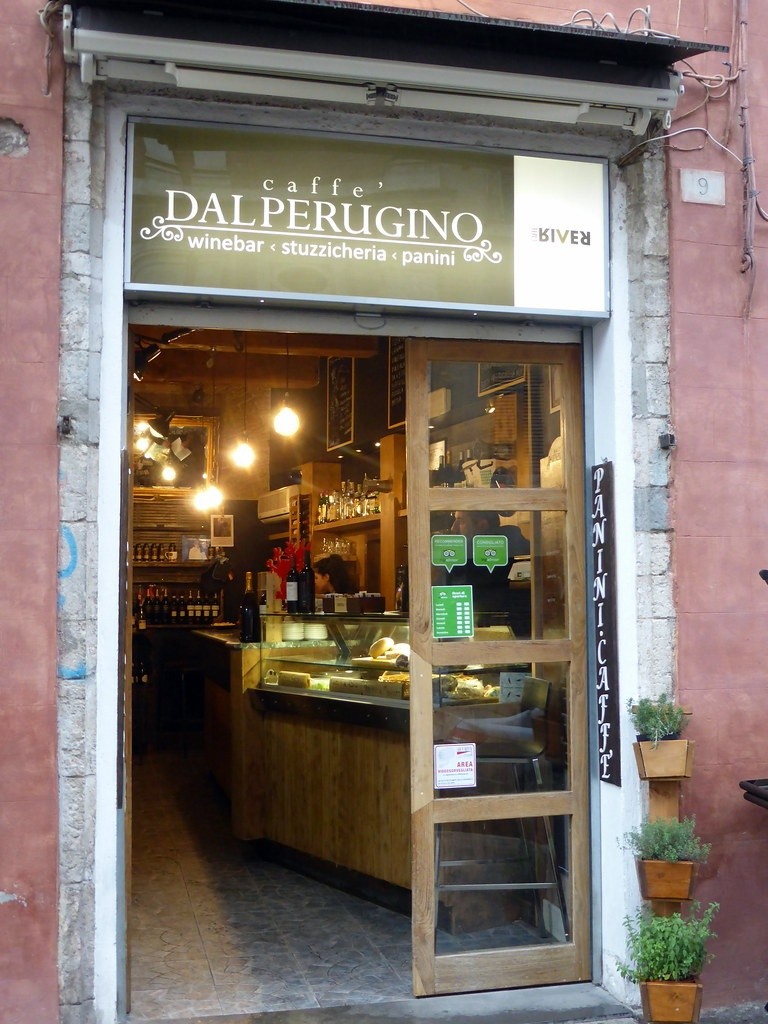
[311,552,359,596]
[436,510,532,639]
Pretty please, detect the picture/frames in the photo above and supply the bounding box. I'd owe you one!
[134,411,219,497]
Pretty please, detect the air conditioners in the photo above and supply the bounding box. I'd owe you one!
[258,485,299,524]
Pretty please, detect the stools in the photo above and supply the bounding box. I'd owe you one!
[434,677,570,944]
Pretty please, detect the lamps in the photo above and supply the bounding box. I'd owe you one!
[133,335,162,381]
[135,393,176,440]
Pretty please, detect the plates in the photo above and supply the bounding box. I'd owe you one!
[282,621,360,641]
[211,624,236,627]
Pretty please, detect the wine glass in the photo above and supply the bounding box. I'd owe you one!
[320,537,351,556]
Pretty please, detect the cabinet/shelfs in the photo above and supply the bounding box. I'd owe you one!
[245,612,529,934]
[132,560,230,629]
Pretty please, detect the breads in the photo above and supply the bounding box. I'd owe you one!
[378,670,500,698]
[370,637,411,660]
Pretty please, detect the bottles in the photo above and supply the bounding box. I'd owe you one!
[240,572,261,643]
[132,661,151,689]
[134,584,220,625]
[292,499,310,535]
[133,542,177,562]
[395,564,409,612]
[401,449,475,509]
[286,549,315,613]
[316,473,381,525]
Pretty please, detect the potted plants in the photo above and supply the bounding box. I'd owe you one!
[614,899,720,1024]
[632,694,696,781]
[615,812,712,899]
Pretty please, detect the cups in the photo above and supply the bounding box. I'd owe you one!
[165,552,177,562]
